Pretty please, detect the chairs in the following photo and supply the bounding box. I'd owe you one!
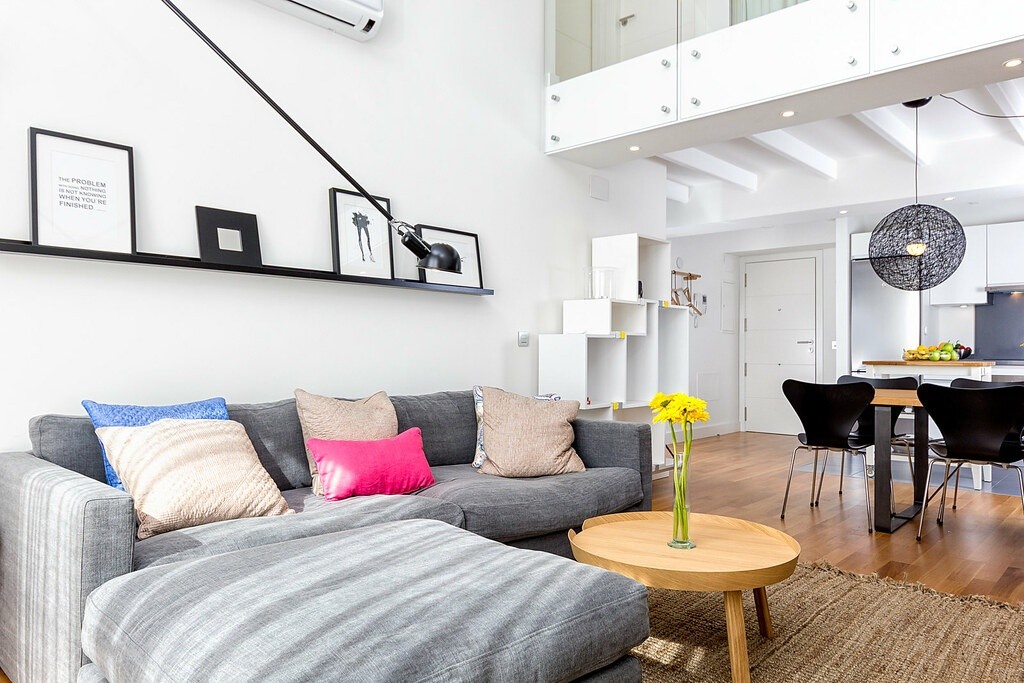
[815,375,919,515]
[781,379,876,533]
[915,384,1024,542]
[936,378,1024,524]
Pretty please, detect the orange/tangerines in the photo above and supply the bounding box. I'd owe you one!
[904,342,946,360]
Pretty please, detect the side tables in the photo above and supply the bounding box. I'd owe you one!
[927,221,1024,306]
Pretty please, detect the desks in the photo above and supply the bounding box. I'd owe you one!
[567,511,801,682]
[866,388,929,533]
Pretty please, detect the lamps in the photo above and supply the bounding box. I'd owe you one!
[869,95,966,291]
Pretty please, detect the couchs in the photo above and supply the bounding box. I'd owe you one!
[0,389,651,683]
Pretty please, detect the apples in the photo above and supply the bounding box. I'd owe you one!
[929,343,959,361]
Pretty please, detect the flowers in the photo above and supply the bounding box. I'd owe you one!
[649,392,711,538]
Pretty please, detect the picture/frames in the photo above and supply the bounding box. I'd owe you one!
[414,224,483,289]
[329,187,394,281]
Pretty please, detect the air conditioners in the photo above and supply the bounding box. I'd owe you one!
[251,0,384,42]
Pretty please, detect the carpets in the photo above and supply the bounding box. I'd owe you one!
[624,559,1024,683]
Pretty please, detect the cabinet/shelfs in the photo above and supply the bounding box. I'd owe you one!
[538,232,690,480]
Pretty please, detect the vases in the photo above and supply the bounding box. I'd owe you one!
[666,452,696,549]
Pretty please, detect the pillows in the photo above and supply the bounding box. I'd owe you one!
[293,388,398,496]
[306,426,435,502]
[95,418,295,540]
[82,396,229,492]
[478,386,586,478]
[471,386,562,469]
